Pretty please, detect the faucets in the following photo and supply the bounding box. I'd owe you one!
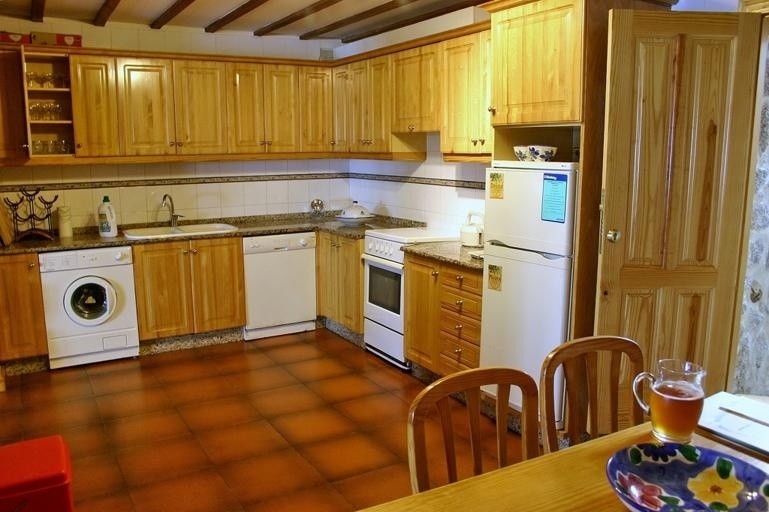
[161,192,185,226]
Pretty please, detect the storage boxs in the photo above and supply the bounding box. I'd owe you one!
[31,30,81,49]
[0,29,33,46]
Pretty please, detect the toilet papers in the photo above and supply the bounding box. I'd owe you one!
[57,205,73,238]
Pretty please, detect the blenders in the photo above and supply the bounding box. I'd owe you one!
[460,210,483,248]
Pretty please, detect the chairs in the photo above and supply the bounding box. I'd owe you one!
[407,362,540,494]
[537,336,646,457]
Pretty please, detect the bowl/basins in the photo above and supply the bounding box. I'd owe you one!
[510,144,559,161]
[335,215,376,227]
[32,140,73,155]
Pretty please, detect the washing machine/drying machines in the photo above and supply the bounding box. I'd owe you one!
[37,245,141,370]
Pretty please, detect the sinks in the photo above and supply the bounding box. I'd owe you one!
[178,223,238,236]
[121,226,185,240]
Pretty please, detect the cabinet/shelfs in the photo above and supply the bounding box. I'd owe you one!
[332,50,427,161]
[391,33,438,137]
[314,231,364,338]
[434,19,491,162]
[401,253,481,399]
[222,55,298,160]
[131,235,247,344]
[0,252,47,371]
[0,43,120,166]
[291,58,337,156]
[477,0,581,129]
[111,50,229,164]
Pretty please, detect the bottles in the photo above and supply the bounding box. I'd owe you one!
[55,205,73,244]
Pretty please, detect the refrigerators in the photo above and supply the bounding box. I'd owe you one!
[476,158,579,431]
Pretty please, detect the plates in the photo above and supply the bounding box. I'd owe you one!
[603,443,767,511]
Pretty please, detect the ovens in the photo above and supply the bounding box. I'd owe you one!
[362,254,412,370]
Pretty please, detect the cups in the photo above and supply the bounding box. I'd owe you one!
[634,353,708,445]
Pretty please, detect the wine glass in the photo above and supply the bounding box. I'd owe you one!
[25,71,70,122]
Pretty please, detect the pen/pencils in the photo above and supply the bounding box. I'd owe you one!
[719,406,769,427]
